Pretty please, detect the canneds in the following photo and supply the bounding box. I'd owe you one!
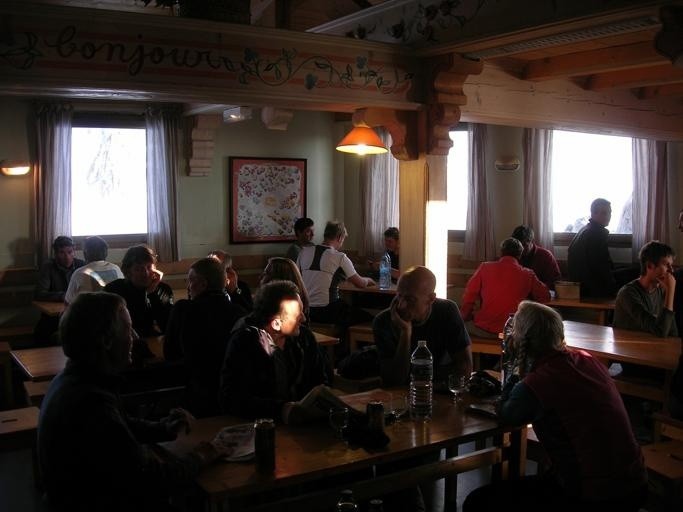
[367,401,384,432]
[254,418,275,472]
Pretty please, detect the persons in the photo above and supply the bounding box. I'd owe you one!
[374,265,474,469]
[293,218,376,379]
[511,223,562,301]
[366,225,401,286]
[284,217,316,267]
[36,292,212,511]
[461,302,647,510]
[457,237,550,340]
[164,250,330,432]
[611,241,679,385]
[43,235,175,361]
[566,197,621,300]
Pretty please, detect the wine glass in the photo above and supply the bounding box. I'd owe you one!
[447,372,465,402]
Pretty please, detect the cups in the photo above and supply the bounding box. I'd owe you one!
[329,408,351,437]
[389,392,409,420]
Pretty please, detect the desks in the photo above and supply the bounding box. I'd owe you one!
[0,249,682,511]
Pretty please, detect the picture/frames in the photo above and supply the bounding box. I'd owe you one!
[227,154,308,245]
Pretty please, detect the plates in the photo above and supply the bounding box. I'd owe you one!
[211,430,256,465]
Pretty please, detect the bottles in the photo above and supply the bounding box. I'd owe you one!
[409,340,433,420]
[499,314,518,391]
[379,252,391,289]
[409,422,430,446]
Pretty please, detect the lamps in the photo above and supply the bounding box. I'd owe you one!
[333,122,389,156]
[0,158,33,177]
